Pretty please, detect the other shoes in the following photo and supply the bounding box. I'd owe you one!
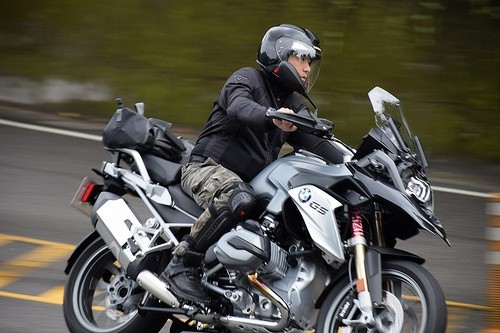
[159,234,208,302]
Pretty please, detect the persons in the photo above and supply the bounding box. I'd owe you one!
[157,23,355,303]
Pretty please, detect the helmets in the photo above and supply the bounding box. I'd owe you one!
[256,23,322,109]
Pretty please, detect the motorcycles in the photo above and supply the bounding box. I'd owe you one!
[64,86,450,333]
